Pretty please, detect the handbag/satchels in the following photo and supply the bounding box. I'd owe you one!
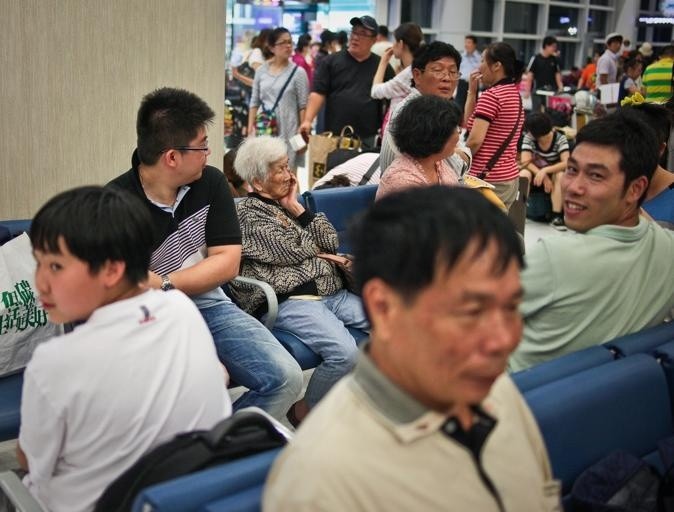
[252,109,278,138]
[306,125,362,187]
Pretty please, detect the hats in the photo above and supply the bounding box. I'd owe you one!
[349,15,379,34]
[638,42,654,58]
[605,32,624,44]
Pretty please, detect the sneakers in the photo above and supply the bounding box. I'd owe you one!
[549,217,568,231]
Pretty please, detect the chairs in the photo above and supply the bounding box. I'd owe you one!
[1,183,674,511]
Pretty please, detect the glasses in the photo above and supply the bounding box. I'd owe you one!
[350,30,373,39]
[160,139,210,153]
[420,68,463,81]
[274,40,294,46]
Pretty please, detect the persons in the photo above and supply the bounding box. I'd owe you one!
[14,15,674,512]
